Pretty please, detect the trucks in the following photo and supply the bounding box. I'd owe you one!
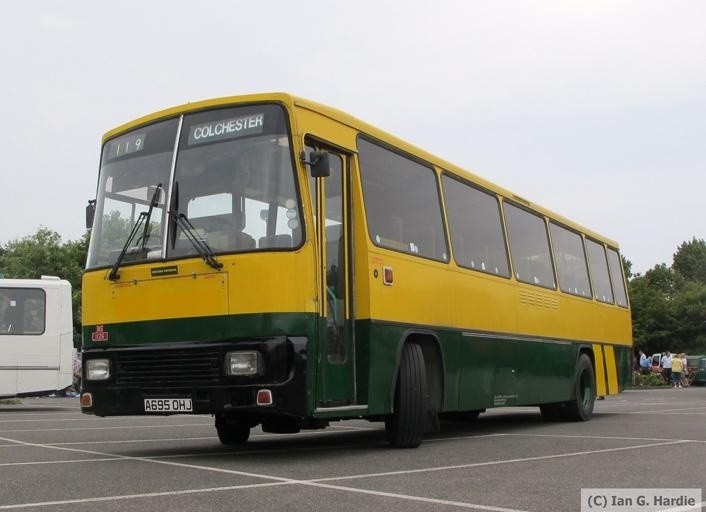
[650,352,706,385]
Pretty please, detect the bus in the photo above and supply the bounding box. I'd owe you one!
[74,90,640,448]
[0,273,75,402]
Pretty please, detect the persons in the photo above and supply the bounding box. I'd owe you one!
[633,348,690,389]
[66,359,82,397]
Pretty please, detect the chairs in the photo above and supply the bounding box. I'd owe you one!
[229,216,436,259]
